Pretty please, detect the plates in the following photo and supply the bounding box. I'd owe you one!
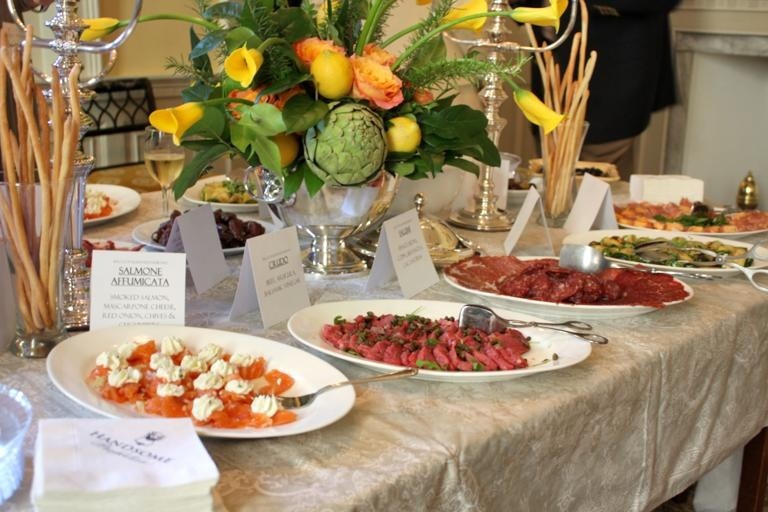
[130,211,280,257]
[611,199,768,240]
[183,172,277,212]
[563,228,766,278]
[443,251,696,322]
[46,323,358,440]
[287,294,593,388]
[528,158,620,188]
[83,181,142,228]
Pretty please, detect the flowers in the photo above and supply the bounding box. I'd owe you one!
[79,0,563,202]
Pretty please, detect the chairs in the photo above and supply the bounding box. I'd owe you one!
[70,77,164,191]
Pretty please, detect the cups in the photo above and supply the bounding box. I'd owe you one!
[0,149,95,361]
[535,111,590,229]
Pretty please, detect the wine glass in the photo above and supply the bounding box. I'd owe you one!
[143,123,189,219]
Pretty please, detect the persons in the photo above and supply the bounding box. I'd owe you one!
[0,0,79,182]
[507,1,685,183]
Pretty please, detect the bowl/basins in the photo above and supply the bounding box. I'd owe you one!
[1,383,34,505]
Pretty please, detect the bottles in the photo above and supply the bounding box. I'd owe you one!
[59,248,98,334]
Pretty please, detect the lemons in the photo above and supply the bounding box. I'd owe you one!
[387,116,422,153]
[275,134,299,168]
[310,50,354,100]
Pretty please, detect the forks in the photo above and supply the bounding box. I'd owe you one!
[270,364,418,409]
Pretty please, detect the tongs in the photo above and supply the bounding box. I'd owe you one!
[628,236,768,295]
[558,243,723,281]
[457,302,609,345]
